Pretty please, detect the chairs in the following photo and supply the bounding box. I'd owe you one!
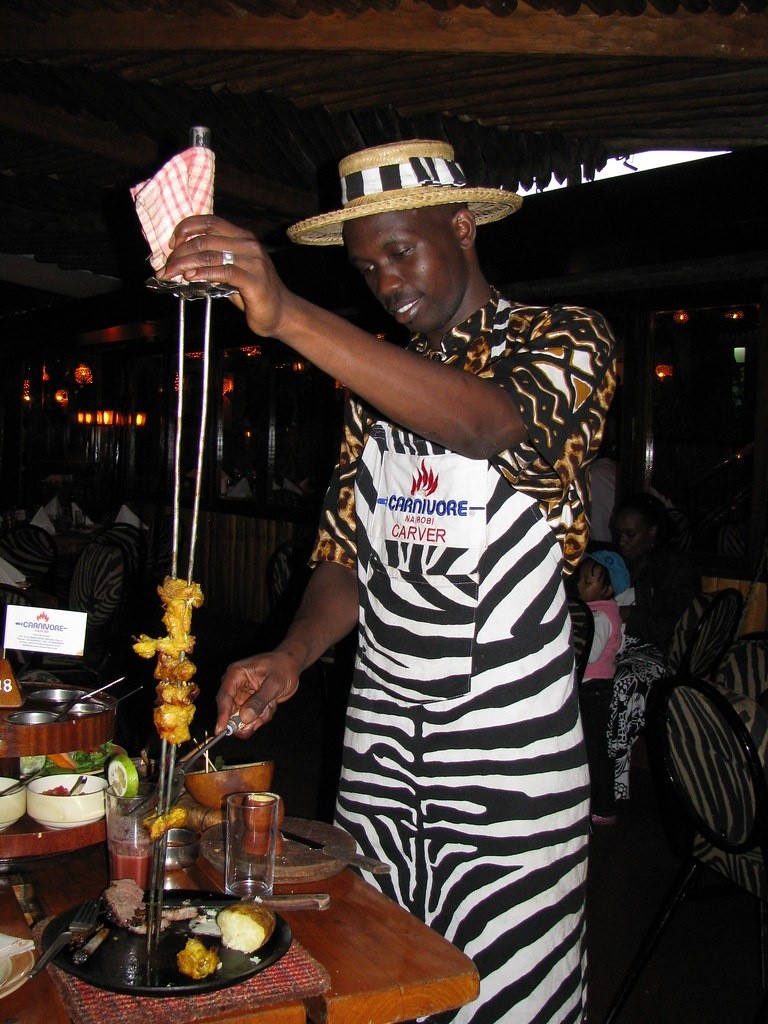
[565,585,768,1024]
[0,511,184,746]
[266,539,336,700]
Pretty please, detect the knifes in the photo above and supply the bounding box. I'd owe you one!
[280,830,391,874]
[162,891,332,911]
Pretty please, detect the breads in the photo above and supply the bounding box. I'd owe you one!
[216,902,275,954]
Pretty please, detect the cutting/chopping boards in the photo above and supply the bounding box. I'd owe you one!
[199,817,357,883]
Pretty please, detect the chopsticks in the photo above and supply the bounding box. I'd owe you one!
[193,731,217,773]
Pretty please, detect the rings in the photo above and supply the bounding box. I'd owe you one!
[221,251,234,265]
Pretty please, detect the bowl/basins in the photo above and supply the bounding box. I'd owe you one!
[183,761,273,810]
[165,827,201,870]
[26,774,108,828]
[2,688,108,728]
[0,777,26,831]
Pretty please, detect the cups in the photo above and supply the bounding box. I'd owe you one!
[226,791,279,896]
[104,780,155,892]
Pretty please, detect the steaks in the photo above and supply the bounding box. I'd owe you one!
[102,877,198,936]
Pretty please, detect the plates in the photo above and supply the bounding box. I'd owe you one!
[78,743,128,774]
[0,932,35,998]
[41,889,293,995]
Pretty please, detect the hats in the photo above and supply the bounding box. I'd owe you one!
[578,549,632,599]
[285,140,524,244]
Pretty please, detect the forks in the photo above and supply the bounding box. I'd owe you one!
[29,898,100,977]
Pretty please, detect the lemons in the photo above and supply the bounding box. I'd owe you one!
[107,754,138,799]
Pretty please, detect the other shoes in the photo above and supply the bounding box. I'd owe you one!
[591,812,618,826]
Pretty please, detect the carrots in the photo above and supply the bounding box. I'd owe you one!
[48,752,76,769]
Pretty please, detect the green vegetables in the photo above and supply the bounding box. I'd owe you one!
[19,740,114,776]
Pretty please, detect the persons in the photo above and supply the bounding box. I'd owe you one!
[588,434,619,542]
[156,137,616,1024]
[599,489,718,805]
[575,550,631,825]
